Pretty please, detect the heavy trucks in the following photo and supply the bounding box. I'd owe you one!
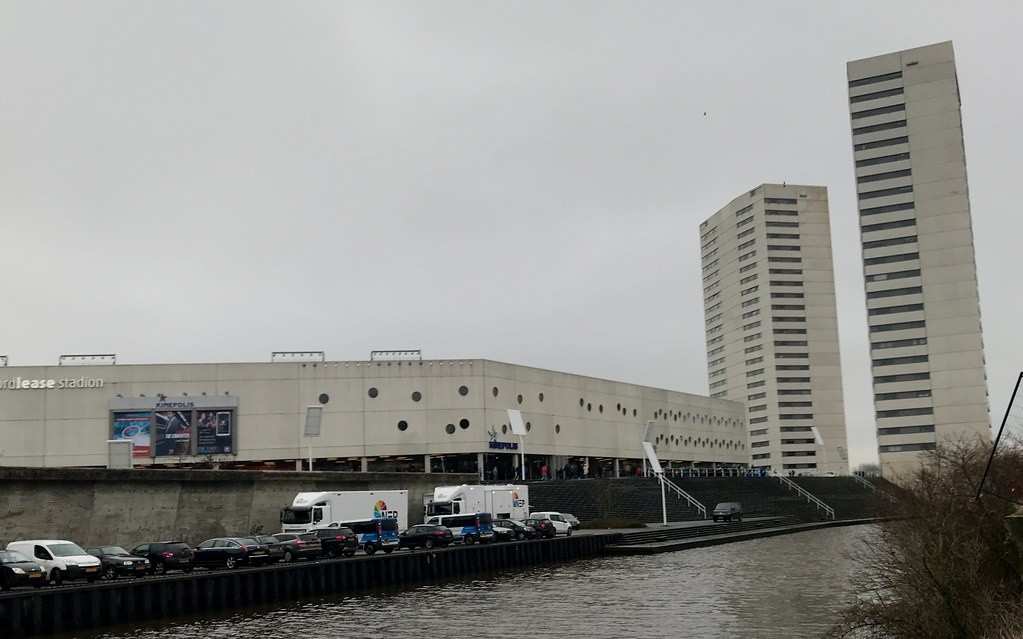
[279,489,409,538]
[424,484,531,523]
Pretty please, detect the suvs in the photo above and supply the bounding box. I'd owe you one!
[4,538,103,585]
[129,539,195,575]
[271,532,323,563]
[394,524,453,549]
[302,526,360,560]
[81,545,150,580]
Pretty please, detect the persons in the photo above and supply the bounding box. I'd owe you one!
[538,463,552,481]
[197,412,216,428]
[562,461,589,480]
[624,462,641,477]
[164,411,183,455]
[510,464,528,480]
[492,466,497,482]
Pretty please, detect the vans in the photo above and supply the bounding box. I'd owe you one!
[712,501,743,522]
[529,511,572,536]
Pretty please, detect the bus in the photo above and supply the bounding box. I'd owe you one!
[425,512,495,546]
[327,517,401,556]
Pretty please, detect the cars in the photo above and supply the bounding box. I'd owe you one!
[245,534,285,566]
[560,513,580,530]
[191,537,268,570]
[490,525,515,543]
[0,549,47,591]
[519,518,556,538]
[492,518,536,541]
